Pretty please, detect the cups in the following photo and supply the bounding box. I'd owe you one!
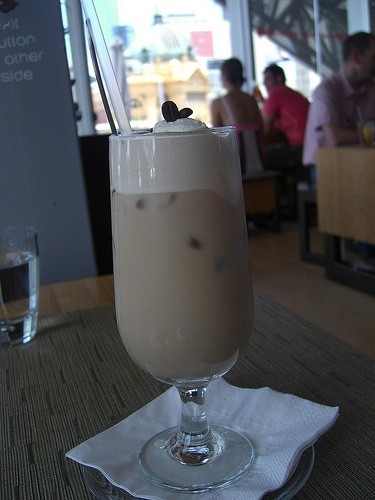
[0,225,40,346]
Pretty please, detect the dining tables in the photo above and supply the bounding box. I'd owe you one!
[0,296,375,500]
[319,145,375,296]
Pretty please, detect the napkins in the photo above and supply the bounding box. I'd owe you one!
[65,377,339,500]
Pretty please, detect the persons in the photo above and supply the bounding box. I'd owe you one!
[252,62,311,199]
[301,31,375,266]
[210,57,264,181]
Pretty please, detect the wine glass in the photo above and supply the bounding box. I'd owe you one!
[110,125,256,494]
